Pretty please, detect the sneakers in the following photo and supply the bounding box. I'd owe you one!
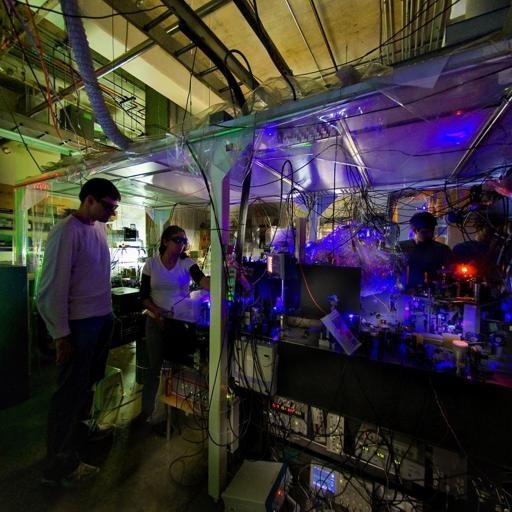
[60,463,100,481]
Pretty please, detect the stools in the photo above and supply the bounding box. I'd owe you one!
[95,365,125,410]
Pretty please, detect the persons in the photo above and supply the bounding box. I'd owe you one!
[396,212,453,290]
[139,225,210,424]
[35,178,120,482]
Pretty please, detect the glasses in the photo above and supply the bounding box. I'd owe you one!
[95,198,118,211]
[167,235,188,245]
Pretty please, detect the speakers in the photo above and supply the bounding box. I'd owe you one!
[1,263,31,410]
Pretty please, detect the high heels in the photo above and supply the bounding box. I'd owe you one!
[130,407,156,424]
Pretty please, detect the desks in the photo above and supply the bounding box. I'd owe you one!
[152,312,511,507]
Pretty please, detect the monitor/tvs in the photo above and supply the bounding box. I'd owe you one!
[294,263,361,327]
